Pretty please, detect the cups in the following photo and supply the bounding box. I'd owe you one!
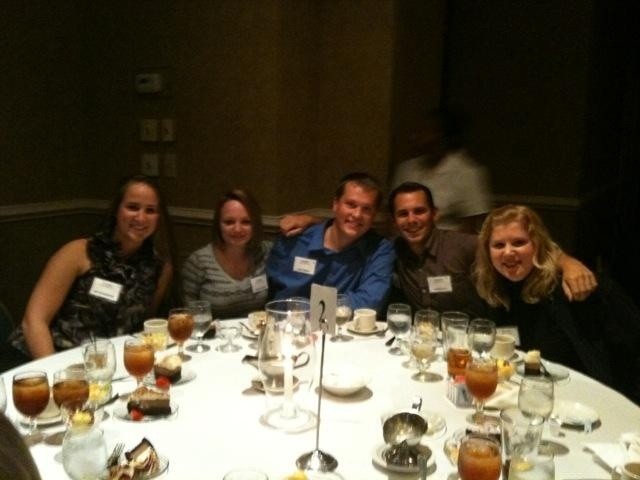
[610,462,640,480]
[144,319,169,361]
[63,425,108,480]
[455,406,555,479]
[353,310,377,330]
[488,333,516,358]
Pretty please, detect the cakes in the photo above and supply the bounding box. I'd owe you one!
[155,354,182,384]
[128,385,171,417]
[525,349,543,376]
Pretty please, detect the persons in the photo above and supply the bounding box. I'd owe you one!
[457,204,620,394]
[279,182,597,325]
[178,189,273,320]
[265,171,396,318]
[2,173,173,367]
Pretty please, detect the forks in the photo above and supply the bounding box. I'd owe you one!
[108,443,126,469]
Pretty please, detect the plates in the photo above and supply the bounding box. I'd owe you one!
[319,372,367,395]
[372,408,446,472]
[348,319,388,336]
[112,403,180,422]
[554,399,595,428]
[144,367,195,386]
[484,350,523,362]
[516,362,569,380]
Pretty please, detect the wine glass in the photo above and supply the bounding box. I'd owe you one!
[13,369,93,444]
[217,326,242,354]
[330,294,353,342]
[169,300,213,362]
[242,298,320,434]
[82,338,154,397]
[385,305,554,461]
